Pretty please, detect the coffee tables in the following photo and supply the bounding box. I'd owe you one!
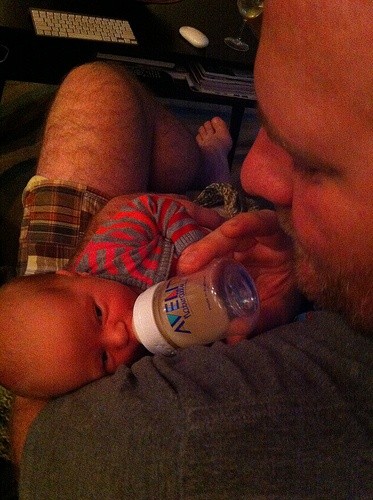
[0,0,258,173]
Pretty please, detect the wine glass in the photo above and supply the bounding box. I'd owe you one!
[224,0,263,51]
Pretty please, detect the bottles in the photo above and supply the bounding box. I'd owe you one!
[132,258,260,354]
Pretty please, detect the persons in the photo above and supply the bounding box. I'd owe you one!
[1,0,373,500]
[0,195,241,399]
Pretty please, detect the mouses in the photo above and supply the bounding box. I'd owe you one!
[179,26,209,48]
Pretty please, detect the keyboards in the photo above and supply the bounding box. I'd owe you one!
[30,8,137,44]
